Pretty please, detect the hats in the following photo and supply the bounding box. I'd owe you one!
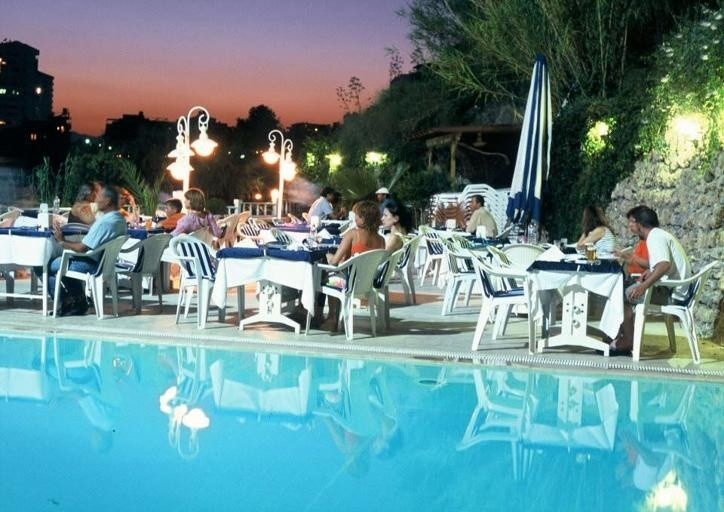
[374,187,390,195]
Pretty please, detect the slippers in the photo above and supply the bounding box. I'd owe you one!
[597,346,633,356]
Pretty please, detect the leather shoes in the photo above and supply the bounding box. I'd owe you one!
[48,301,89,320]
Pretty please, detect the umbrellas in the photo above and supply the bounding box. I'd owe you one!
[504,54,555,244]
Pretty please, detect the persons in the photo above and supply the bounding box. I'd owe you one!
[612,204,654,280]
[31,177,224,316]
[371,416,409,464]
[575,203,620,260]
[308,199,412,323]
[37,348,118,459]
[313,198,386,333]
[619,449,676,502]
[326,192,348,220]
[465,195,498,240]
[373,186,399,219]
[616,209,693,353]
[307,186,346,235]
[621,434,641,478]
[312,404,371,485]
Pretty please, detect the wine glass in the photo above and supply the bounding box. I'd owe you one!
[315,234,323,251]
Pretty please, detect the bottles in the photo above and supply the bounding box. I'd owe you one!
[40,203,50,231]
[585,232,594,256]
[528,223,548,244]
[155,215,159,223]
[52,196,60,228]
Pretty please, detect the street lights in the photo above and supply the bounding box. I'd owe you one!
[155,346,212,461]
[262,125,298,220]
[165,101,219,216]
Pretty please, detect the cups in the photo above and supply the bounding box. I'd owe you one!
[145,216,153,228]
[554,238,567,247]
[587,247,597,261]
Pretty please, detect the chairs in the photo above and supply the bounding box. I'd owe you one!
[1,329,103,406]
[394,184,644,316]
[176,345,399,438]
[486,244,529,336]
[169,232,245,328]
[373,248,406,332]
[465,247,552,351]
[0,203,405,307]
[458,358,668,452]
[306,249,388,342]
[632,259,720,363]
[629,378,703,471]
[107,233,173,318]
[52,235,131,321]
